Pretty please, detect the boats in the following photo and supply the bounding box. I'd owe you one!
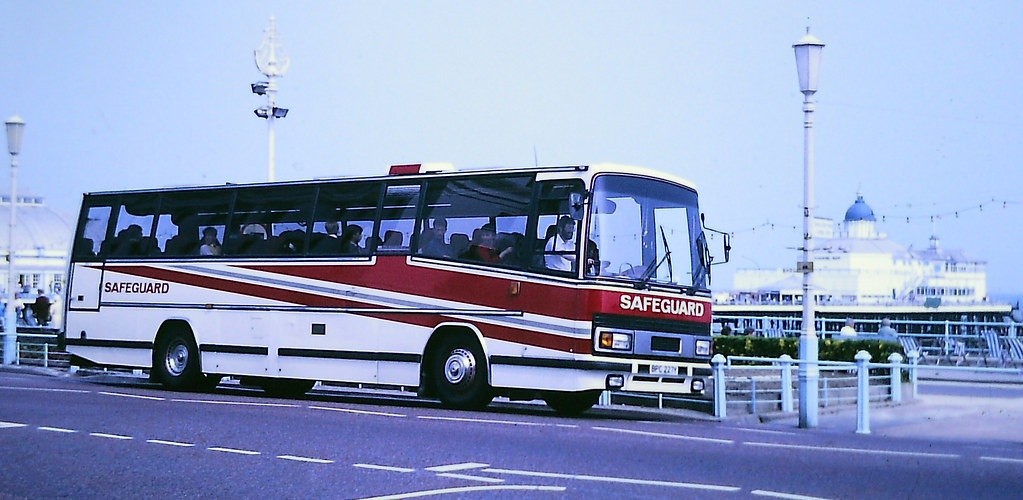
[712,196,1023,366]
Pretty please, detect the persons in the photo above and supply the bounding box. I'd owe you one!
[10,214,760,367]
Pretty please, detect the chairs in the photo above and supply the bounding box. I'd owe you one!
[80,220,558,269]
[898,328,1023,377]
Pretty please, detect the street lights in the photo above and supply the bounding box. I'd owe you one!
[3,115,25,365]
[790,24,819,428]
[251,16,289,183]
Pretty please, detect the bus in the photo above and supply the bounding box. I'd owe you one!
[57,163,731,414]
